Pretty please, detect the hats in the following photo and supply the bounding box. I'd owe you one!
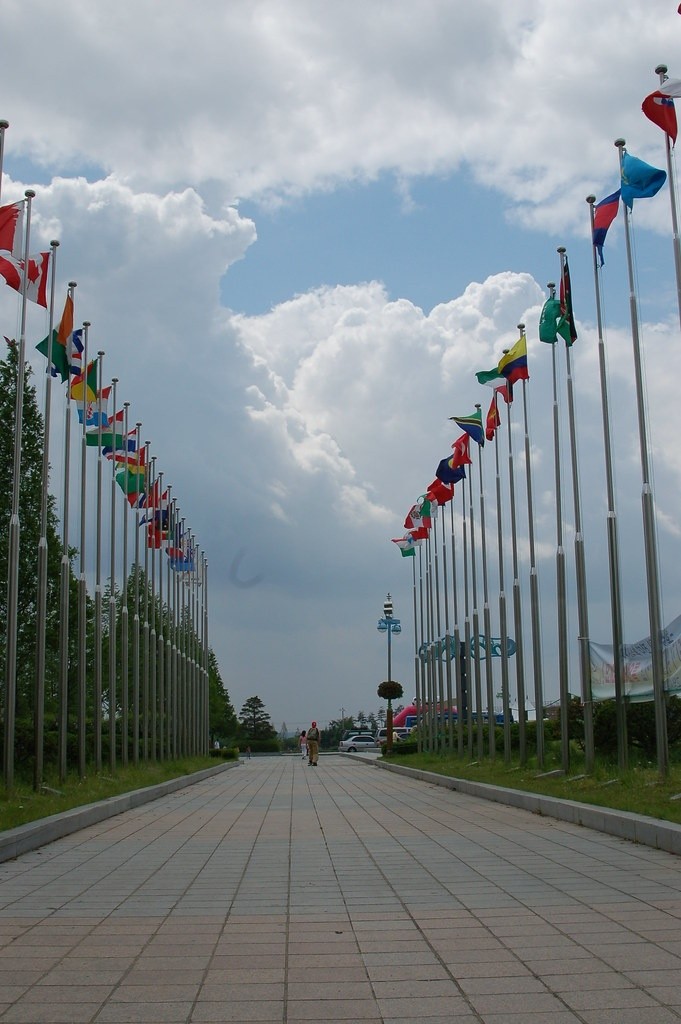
[312,722,316,727]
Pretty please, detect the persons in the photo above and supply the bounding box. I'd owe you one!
[298,721,320,766]
[214,739,220,749]
[246,746,251,759]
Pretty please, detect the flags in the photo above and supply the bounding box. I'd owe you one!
[539,253,578,347]
[0,199,205,588]
[642,78,681,148]
[593,151,667,267]
[391,333,529,556]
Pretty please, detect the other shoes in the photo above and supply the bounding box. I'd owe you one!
[312,762,317,766]
[308,763,312,765]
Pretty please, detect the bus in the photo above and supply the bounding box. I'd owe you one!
[405,711,517,727]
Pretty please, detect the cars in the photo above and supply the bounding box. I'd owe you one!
[337,735,381,753]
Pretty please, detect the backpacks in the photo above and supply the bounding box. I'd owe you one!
[308,728,317,739]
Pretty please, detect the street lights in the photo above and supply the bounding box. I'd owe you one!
[376,592,402,746]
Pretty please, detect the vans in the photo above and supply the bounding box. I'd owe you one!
[375,727,412,746]
[342,729,377,741]
